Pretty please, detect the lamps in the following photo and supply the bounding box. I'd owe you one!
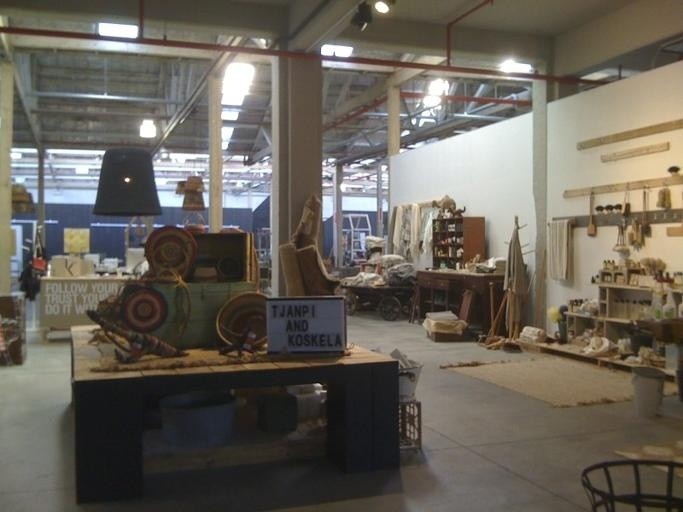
[351,0,372,32]
[375,0,394,15]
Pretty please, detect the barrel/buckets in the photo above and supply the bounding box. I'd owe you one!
[216,290,268,346]
[398,359,422,403]
[632,365,667,419]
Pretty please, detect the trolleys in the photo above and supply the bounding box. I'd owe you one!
[340,280,413,320]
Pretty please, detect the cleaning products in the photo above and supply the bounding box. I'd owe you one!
[662,278,677,320]
[650,276,664,322]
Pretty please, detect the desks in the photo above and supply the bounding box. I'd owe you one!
[40,274,146,343]
[70,325,400,506]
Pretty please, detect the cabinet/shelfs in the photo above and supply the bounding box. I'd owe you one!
[409,270,504,324]
[538,268,683,379]
[432,217,486,268]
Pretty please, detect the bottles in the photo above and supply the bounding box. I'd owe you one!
[653,282,663,320]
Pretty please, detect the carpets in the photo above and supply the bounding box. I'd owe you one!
[440,353,683,409]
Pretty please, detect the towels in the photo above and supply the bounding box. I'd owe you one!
[546,220,571,281]
